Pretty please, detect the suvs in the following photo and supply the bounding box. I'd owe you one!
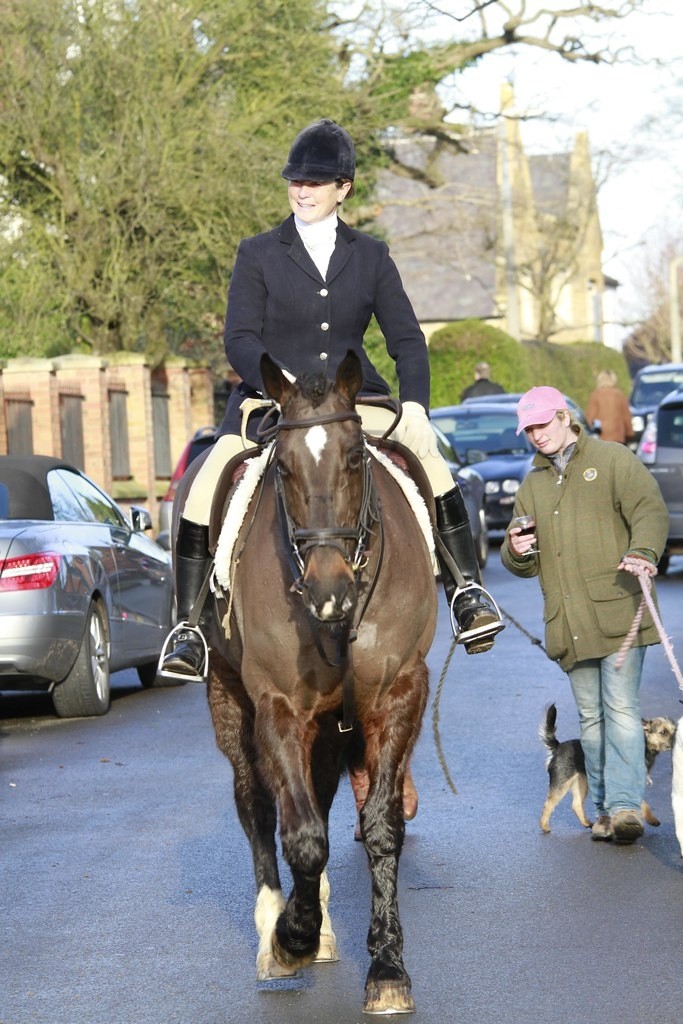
[637,385,683,579]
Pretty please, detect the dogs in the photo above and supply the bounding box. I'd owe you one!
[539,701,677,836]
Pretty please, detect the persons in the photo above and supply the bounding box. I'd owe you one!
[162,122,499,677]
[500,386,670,844]
[461,363,504,402]
[587,370,635,444]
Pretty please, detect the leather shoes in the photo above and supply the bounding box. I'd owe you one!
[166,514,211,675]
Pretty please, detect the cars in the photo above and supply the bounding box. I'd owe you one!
[427,395,605,544]
[0,455,174,719]
[628,364,683,455]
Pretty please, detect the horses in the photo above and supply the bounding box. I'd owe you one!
[202,349,440,1016]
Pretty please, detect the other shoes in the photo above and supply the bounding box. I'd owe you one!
[591,815,612,840]
[612,810,644,845]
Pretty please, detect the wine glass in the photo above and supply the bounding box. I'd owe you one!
[514,516,541,556]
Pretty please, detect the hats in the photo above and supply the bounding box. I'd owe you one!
[515,386,569,437]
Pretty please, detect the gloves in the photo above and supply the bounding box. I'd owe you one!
[390,402,438,458]
[270,369,297,413]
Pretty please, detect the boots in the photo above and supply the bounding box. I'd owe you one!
[348,761,371,840]
[401,764,418,819]
[431,486,500,655]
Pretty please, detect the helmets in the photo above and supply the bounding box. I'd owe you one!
[282,118,356,182]
